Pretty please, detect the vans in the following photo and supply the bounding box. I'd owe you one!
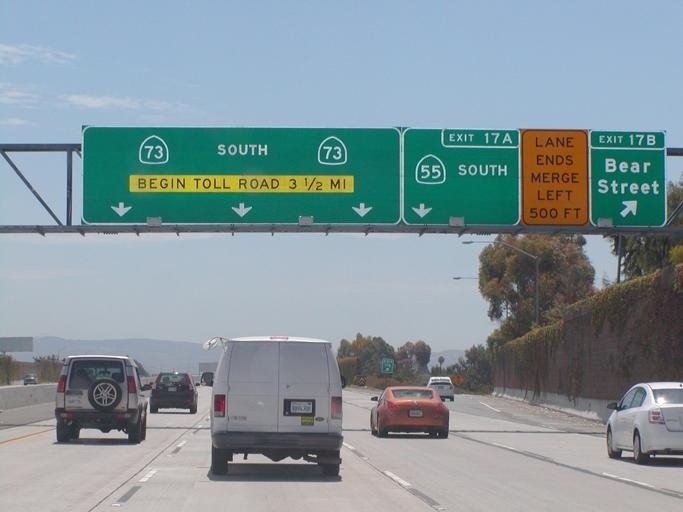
[199,334,347,475]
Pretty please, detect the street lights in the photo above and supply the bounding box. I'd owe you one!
[461,240,540,325]
[452,277,511,338]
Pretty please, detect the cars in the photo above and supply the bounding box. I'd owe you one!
[22,372,38,385]
[369,385,455,438]
[427,375,454,401]
[604,381,682,464]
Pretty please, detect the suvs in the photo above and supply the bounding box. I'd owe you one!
[146,371,201,413]
[54,354,152,444]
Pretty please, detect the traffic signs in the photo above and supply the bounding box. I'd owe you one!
[400,127,521,224]
[380,358,394,373]
[82,124,401,223]
[589,130,666,227]
